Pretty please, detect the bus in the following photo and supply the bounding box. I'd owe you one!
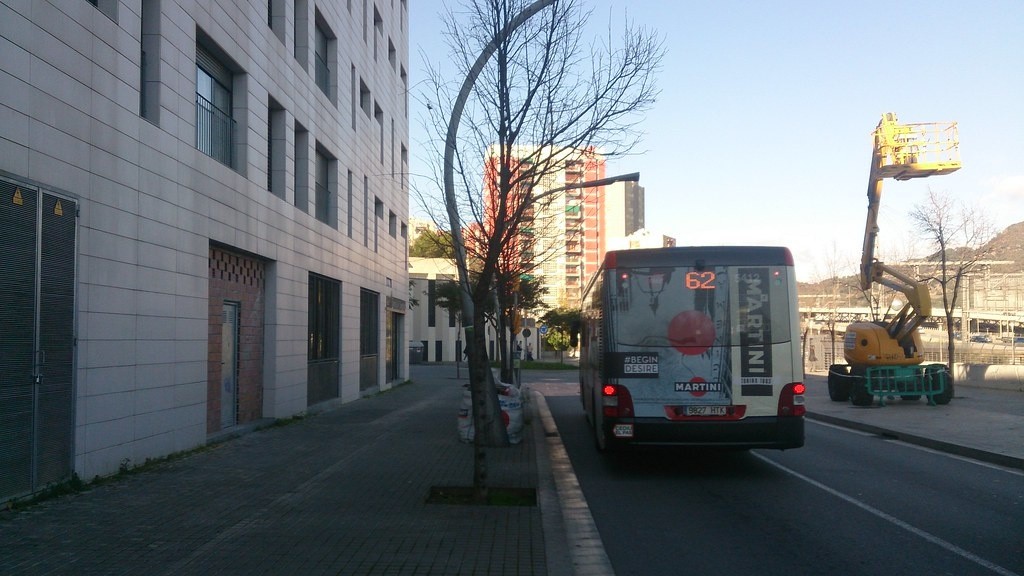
[569,245,806,463]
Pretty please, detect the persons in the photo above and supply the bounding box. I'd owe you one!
[527,343,534,360]
[516,340,523,351]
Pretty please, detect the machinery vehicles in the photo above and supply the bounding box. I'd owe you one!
[827,112,963,406]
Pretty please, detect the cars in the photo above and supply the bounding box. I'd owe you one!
[953,331,962,340]
[970,335,986,342]
[1014,338,1024,343]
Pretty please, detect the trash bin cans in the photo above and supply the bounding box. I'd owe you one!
[409,341,425,363]
